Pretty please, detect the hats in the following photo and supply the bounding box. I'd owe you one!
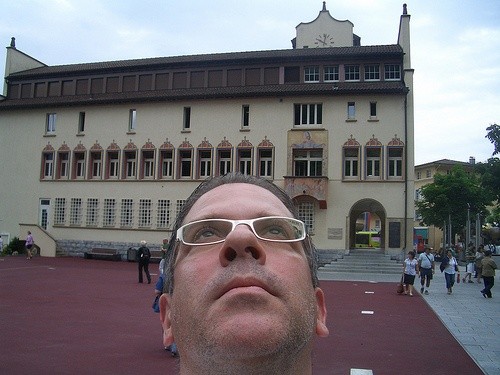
[141,240,147,245]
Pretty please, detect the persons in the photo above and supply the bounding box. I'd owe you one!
[25,231,33,260]
[434,238,498,298]
[402,252,419,296]
[137,240,151,284]
[416,244,435,295]
[0,236,3,251]
[152,174,329,375]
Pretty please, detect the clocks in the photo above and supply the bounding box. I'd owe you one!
[314,33,334,48]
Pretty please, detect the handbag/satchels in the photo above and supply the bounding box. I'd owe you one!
[454,265,458,271]
[396,276,404,293]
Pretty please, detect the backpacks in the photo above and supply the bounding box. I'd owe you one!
[140,247,149,261]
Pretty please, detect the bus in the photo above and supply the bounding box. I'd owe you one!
[354,230,382,248]
[482,227,500,256]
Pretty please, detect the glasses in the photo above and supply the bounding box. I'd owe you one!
[172,216,306,246]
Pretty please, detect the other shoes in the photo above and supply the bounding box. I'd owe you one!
[425,290,429,295]
[420,288,424,293]
[410,292,413,295]
[447,292,452,295]
[485,291,492,298]
[480,290,486,298]
[468,280,473,283]
[405,291,408,294]
[167,347,179,357]
[462,278,466,282]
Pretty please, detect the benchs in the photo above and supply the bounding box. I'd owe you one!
[84,248,120,262]
[150,251,162,263]
